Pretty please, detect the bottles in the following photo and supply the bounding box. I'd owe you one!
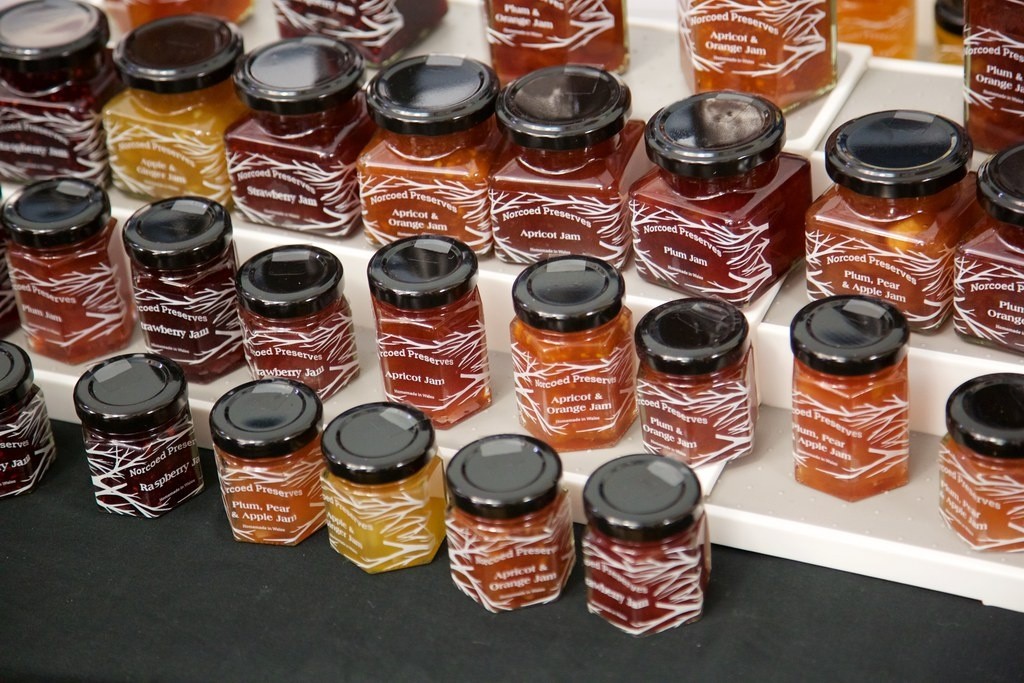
[318,403,447,575]
[209,380,327,547]
[1,0,1024,361]
[633,296,758,464]
[789,294,910,502]
[234,243,362,404]
[367,233,492,430]
[936,373,1024,556]
[582,455,711,636]
[440,435,576,613]
[507,255,636,453]
[121,195,245,386]
[74,352,203,519]
[1,340,55,496]
